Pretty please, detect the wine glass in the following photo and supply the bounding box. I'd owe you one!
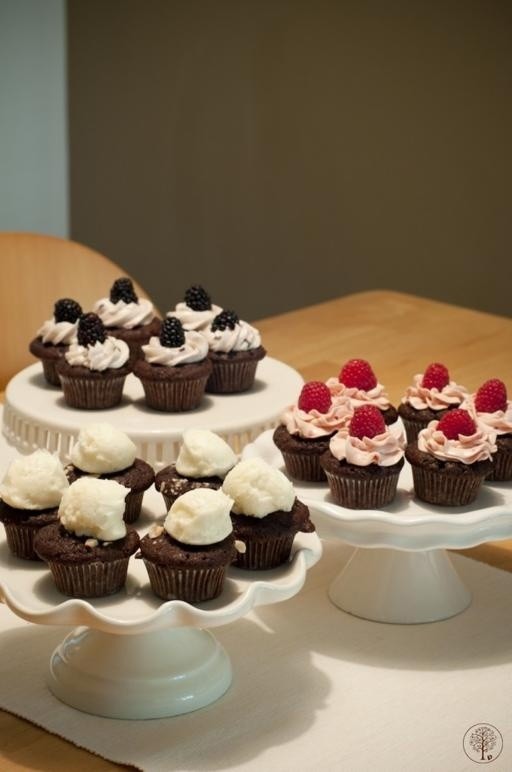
[0,354,502,735]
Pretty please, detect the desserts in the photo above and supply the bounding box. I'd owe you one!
[270,354,512,510]
[0,425,316,604]
[28,278,268,414]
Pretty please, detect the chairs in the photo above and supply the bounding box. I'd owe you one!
[3,231,157,404]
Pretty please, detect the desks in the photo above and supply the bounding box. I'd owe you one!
[2,286,509,770]
